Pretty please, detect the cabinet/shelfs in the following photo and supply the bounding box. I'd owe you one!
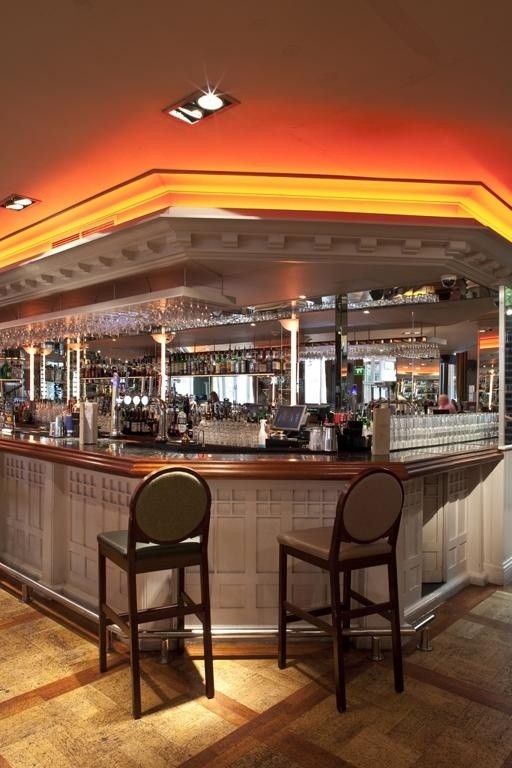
[0,281,500,455]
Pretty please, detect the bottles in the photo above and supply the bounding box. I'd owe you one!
[166,399,188,438]
[170,348,286,375]
[125,409,159,433]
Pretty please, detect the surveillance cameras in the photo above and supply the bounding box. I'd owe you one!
[440,275,457,289]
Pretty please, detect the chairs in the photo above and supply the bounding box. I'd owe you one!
[277,467,405,713]
[97,466,215,718]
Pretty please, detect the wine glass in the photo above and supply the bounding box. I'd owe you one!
[401,342,440,359]
[92,300,218,335]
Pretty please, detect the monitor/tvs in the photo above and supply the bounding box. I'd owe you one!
[271,404,307,431]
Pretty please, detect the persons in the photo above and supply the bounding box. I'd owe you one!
[208,391,220,403]
[436,394,457,413]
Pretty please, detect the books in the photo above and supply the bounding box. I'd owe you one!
[79,402,98,445]
